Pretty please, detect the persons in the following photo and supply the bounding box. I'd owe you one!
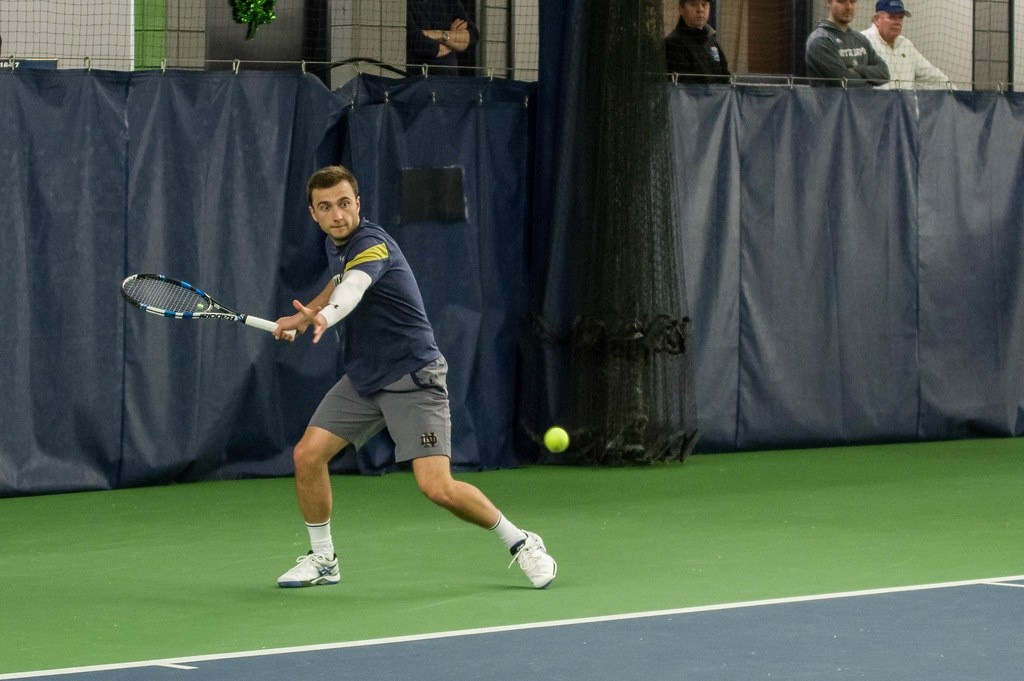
[858,0,958,91]
[404,0,479,79]
[663,0,732,86]
[271,164,557,589]
[803,0,891,90]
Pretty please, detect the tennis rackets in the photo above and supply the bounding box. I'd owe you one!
[119,273,298,342]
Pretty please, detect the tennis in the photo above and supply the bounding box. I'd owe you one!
[544,426,569,453]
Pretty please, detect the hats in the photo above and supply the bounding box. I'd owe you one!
[876,0,911,18]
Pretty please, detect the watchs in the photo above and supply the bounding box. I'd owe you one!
[441,30,449,44]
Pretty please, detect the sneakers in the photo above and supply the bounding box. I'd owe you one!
[508,530,557,588]
[278,550,340,587]
